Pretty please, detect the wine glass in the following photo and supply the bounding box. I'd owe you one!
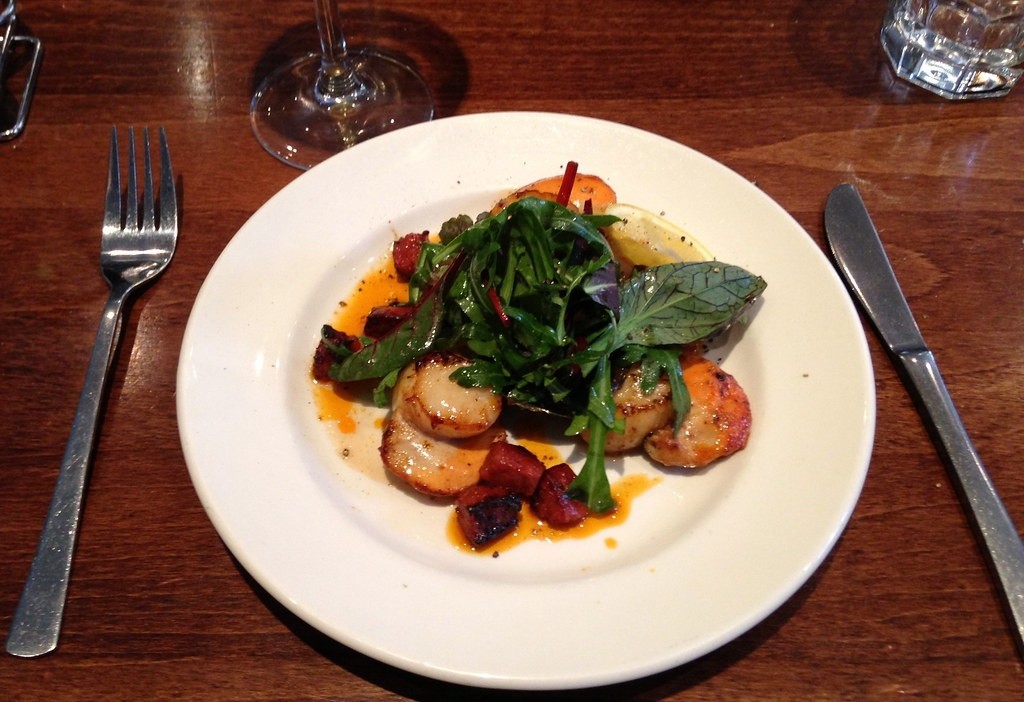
[249,0,434,171]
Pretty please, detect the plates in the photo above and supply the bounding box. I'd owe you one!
[176,111,877,690]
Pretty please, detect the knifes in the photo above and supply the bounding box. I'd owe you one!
[820,184,1024,644]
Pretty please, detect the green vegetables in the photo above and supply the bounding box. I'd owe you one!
[337,198,767,512]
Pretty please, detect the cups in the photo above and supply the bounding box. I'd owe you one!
[881,1,1023,100]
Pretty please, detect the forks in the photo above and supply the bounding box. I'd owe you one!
[6,126,178,657]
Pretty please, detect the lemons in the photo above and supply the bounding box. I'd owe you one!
[603,205,711,270]
